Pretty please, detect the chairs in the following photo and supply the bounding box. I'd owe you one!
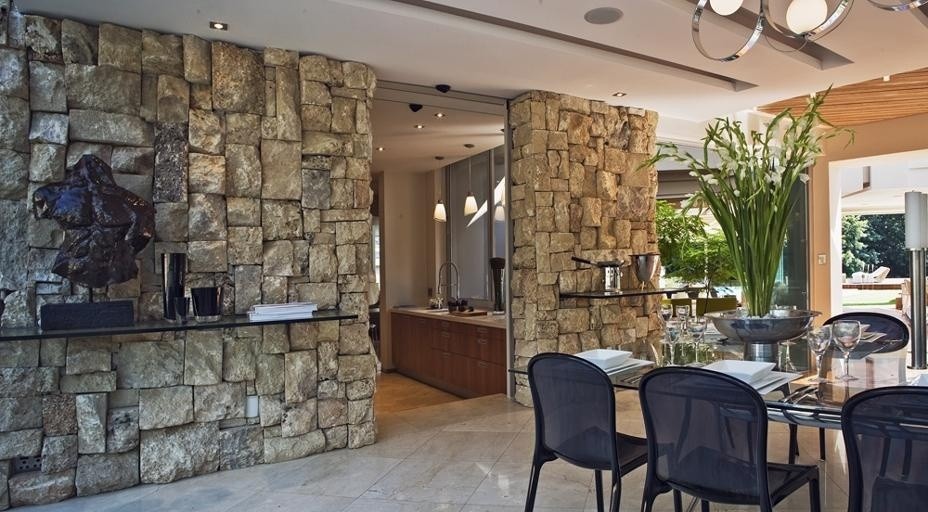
[638,366,821,512]
[524,351,683,511]
[660,298,695,318]
[695,297,737,318]
[674,337,813,462]
[790,313,912,479]
[853,266,891,282]
[841,387,928,512]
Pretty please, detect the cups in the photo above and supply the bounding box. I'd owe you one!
[161,253,221,325]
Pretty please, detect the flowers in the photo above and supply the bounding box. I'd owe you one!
[634,82,855,319]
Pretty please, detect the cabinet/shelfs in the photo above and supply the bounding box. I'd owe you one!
[388,313,507,399]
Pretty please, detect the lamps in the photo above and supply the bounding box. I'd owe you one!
[433,155,448,222]
[904,191,927,370]
[463,144,479,216]
[692,0,926,62]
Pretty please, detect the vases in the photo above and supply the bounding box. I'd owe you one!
[705,307,823,370]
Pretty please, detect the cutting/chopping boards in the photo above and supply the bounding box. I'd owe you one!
[451,310,487,317]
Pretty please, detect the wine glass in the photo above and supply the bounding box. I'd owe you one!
[809,320,861,381]
[660,304,723,366]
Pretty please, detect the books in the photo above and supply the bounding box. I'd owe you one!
[246,309,314,323]
[251,301,319,314]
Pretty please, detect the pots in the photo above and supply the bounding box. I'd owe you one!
[571,255,625,268]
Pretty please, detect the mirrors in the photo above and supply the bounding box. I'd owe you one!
[490,144,505,311]
[445,149,491,308]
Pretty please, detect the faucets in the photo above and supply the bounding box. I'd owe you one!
[438,262,459,304]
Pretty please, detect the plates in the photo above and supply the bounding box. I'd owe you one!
[571,347,655,377]
[698,358,804,396]
[797,324,887,346]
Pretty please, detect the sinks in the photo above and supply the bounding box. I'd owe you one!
[426,308,449,313]
